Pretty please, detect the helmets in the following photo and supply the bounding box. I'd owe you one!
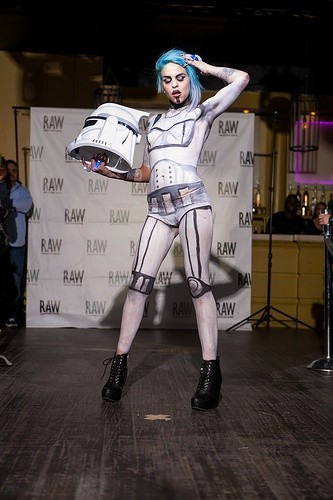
[65,103,149,173]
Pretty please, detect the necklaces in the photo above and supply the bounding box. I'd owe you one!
[164,106,189,119]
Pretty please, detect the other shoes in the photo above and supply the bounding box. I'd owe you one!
[6,317,17,328]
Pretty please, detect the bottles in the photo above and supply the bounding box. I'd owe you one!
[287,182,333,217]
[254,183,262,207]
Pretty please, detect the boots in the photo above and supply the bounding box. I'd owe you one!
[191,356,222,411]
[100,352,128,403]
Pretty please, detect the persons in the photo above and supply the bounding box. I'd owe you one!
[0,153,33,329]
[80,50,252,412]
[267,193,333,235]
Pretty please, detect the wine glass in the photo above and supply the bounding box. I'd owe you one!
[318,209,329,239]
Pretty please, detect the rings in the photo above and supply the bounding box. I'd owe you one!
[83,164,91,172]
[190,54,202,62]
